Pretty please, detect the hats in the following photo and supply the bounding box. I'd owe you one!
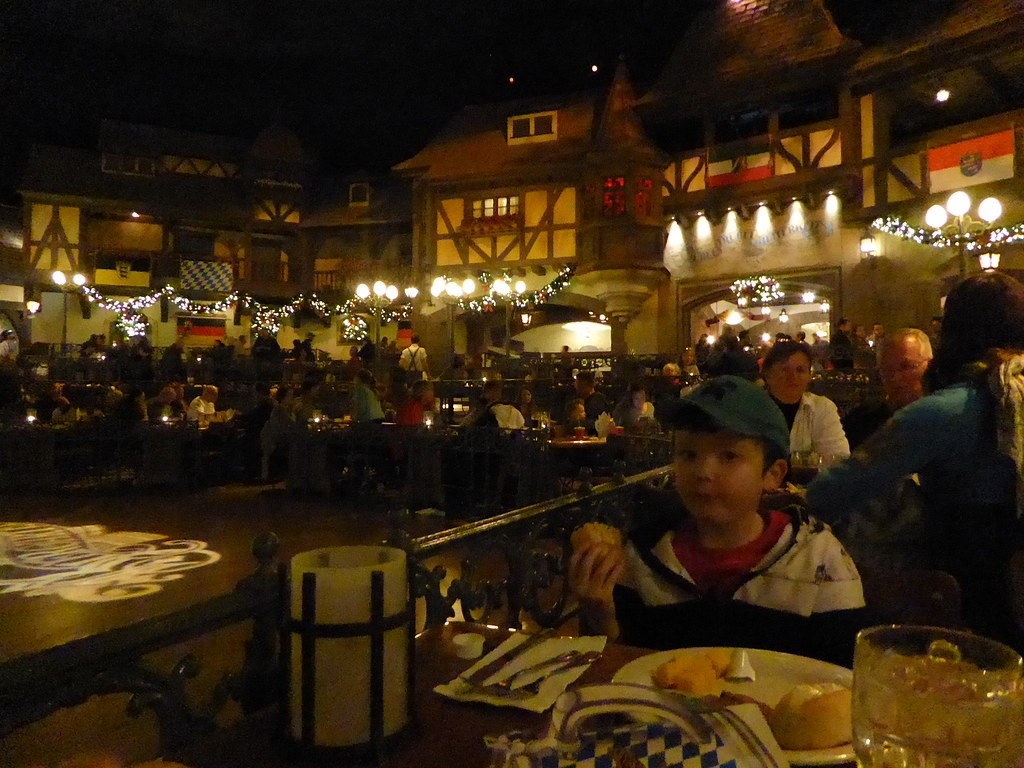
[654,373,791,457]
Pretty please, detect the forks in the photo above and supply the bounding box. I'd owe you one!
[474,651,602,700]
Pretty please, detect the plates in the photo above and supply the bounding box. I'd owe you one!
[613,646,856,764]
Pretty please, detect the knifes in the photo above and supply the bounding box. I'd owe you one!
[469,627,560,685]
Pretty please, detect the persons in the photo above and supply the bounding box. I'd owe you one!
[288,332,315,361]
[562,373,864,669]
[556,344,699,435]
[775,316,887,374]
[201,327,285,381]
[928,316,944,352]
[399,332,434,382]
[0,329,235,488]
[804,273,1023,653]
[347,335,399,366]
[841,326,933,450]
[459,378,543,430]
[236,366,443,509]
[760,340,852,473]
[696,327,758,382]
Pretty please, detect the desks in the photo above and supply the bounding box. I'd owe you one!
[379,422,607,447]
[166,622,661,768]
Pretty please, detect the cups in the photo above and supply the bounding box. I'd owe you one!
[532,412,551,432]
[851,624,1024,768]
[575,427,586,440]
[791,450,831,474]
[453,633,485,660]
[616,426,624,436]
[312,410,322,424]
[423,410,433,426]
[291,546,413,745]
[27,407,38,424]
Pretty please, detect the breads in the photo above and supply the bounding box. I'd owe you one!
[656,652,724,697]
[572,523,622,569]
[703,683,853,750]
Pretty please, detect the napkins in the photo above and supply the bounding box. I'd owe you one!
[431,632,607,713]
